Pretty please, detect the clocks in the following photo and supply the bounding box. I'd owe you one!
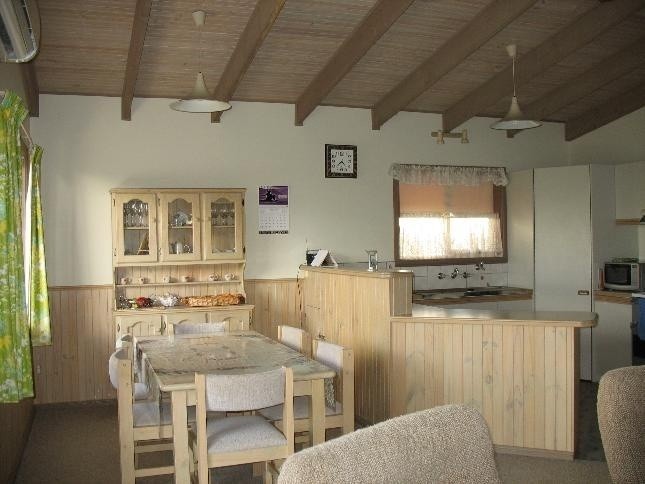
[325,144,357,177]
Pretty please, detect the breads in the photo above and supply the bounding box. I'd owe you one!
[188,294,239,307]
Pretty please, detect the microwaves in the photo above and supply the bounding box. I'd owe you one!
[604,262,645,291]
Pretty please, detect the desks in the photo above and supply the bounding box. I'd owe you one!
[134,329,337,484]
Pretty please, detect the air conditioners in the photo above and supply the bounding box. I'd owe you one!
[0,0,42,64]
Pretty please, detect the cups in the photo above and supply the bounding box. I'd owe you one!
[116,272,237,283]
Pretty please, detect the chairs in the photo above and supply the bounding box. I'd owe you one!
[277,404,501,484]
[168,323,232,338]
[596,365,645,484]
[116,333,177,405]
[273,324,312,356]
[186,365,297,483]
[111,341,192,483]
[249,337,356,484]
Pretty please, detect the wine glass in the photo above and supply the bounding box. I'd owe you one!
[211,200,234,226]
[123,200,148,227]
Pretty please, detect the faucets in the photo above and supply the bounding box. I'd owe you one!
[451,268,459,278]
[475,259,486,271]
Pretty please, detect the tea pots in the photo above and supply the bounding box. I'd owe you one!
[158,293,178,307]
[168,238,190,254]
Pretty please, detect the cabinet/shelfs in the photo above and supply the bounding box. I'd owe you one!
[111,188,254,379]
[592,300,632,383]
[615,162,645,225]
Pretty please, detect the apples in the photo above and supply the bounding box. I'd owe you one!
[145,298,151,306]
[137,296,146,307]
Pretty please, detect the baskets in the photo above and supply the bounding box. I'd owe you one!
[188,294,239,306]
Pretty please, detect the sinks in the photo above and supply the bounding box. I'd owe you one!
[416,291,465,299]
[465,289,525,297]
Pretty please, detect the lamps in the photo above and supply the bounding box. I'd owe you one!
[169,10,232,113]
[490,43,543,131]
